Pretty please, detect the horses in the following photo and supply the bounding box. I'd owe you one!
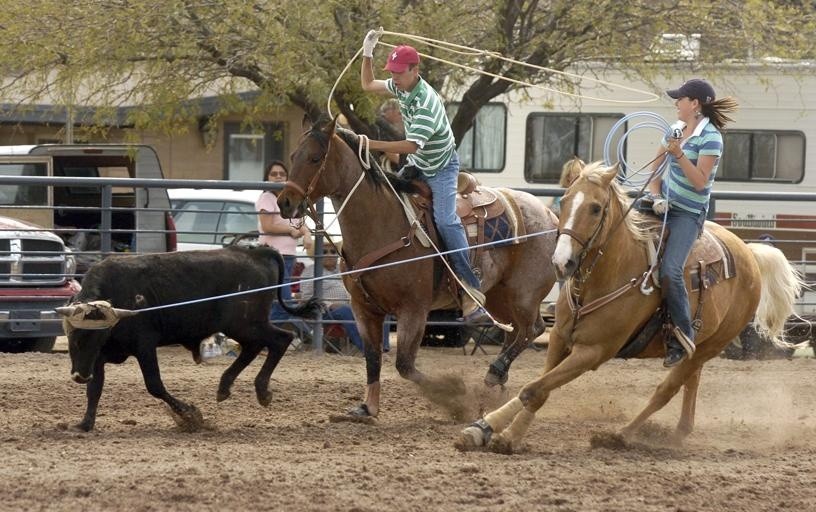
[452,155,810,458]
[278,113,562,422]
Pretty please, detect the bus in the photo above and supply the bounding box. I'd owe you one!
[333,62,816,325]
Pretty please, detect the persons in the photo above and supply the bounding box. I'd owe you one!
[301,233,391,355]
[628,79,740,368]
[367,98,407,179]
[338,25,484,317]
[256,160,310,331]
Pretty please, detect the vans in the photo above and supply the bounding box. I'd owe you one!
[169,187,391,351]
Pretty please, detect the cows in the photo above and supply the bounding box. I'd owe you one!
[52,242,325,432]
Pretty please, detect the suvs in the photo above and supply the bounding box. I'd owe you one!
[0,217,83,354]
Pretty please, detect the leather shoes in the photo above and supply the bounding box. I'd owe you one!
[663,339,697,367]
[463,291,487,317]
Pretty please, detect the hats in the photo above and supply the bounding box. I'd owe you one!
[382,46,420,71]
[667,78,716,102]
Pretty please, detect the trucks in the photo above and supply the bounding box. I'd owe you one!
[0,145,176,278]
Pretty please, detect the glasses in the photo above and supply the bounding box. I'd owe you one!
[270,170,287,176]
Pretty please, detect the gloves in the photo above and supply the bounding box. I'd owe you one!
[361,27,383,57]
